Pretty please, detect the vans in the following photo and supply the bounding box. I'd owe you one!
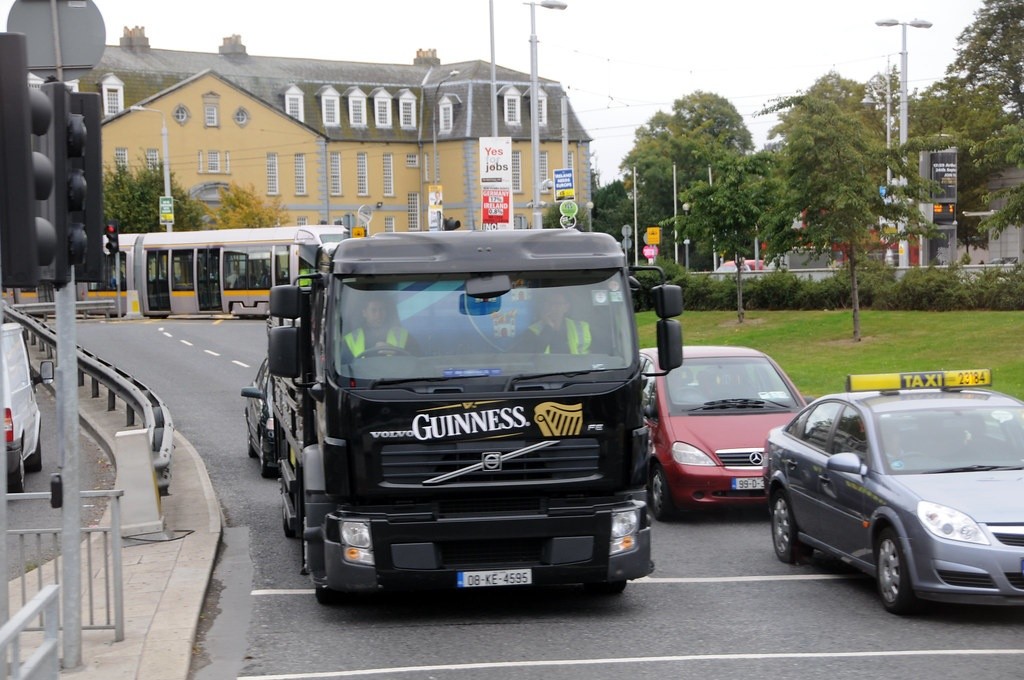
[1,320,56,493]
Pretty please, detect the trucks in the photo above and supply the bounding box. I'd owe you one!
[269,227,687,608]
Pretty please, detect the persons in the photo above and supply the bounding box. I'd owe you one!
[101,259,290,297]
[434,191,442,206]
[338,291,421,363]
[518,285,595,355]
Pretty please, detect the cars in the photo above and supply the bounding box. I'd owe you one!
[632,342,816,520]
[239,357,280,477]
[762,369,1023,615]
[714,259,762,272]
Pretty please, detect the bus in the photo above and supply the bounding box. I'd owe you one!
[1,224,354,319]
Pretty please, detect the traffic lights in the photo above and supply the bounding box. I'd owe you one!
[42,78,87,280]
[106,220,118,252]
[443,217,462,231]
[0,30,58,287]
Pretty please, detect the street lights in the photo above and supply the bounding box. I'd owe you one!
[529,0,567,226]
[877,18,934,266]
[130,104,175,233]
[431,69,460,185]
